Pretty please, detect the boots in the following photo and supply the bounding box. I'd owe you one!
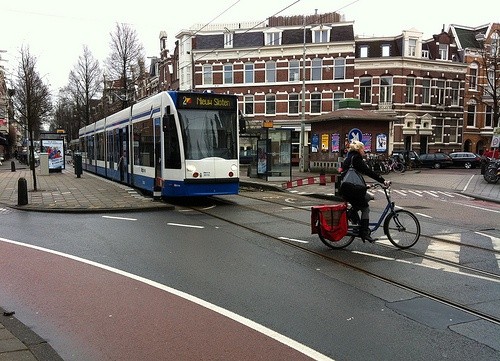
[350,212,360,225]
[360,218,379,243]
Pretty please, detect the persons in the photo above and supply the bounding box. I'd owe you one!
[339,140,390,243]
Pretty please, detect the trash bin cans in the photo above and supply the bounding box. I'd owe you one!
[74,153,82,175]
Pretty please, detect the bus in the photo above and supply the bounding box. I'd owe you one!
[69,90,246,197]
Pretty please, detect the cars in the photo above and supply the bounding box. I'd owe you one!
[401,150,481,170]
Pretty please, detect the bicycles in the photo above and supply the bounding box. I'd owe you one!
[363,153,405,175]
[310,179,421,250]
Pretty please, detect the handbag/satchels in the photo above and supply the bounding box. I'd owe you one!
[338,155,367,195]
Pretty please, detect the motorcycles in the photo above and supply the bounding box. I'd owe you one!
[481,157,500,184]
